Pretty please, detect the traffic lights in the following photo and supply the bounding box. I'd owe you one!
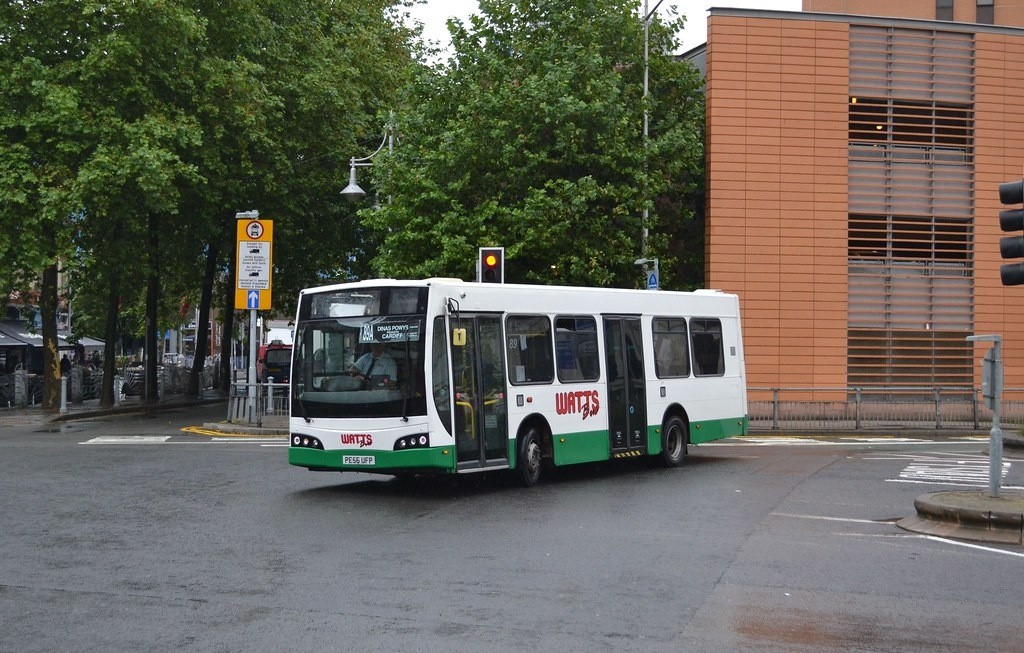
[998,177,1024,289]
[478,247,506,285]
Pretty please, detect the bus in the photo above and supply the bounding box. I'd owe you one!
[287,277,750,489]
[257,340,297,385]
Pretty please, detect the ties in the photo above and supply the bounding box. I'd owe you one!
[364,358,378,380]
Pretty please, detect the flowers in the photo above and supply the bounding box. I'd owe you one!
[115,354,136,368]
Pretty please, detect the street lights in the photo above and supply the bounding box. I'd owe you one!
[340,108,398,254]
[635,257,660,290]
[234,210,262,423]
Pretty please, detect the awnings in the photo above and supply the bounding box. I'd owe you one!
[0,321,105,350]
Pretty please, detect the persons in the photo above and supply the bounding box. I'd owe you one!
[92,351,102,368]
[349,337,398,387]
[61,354,71,376]
